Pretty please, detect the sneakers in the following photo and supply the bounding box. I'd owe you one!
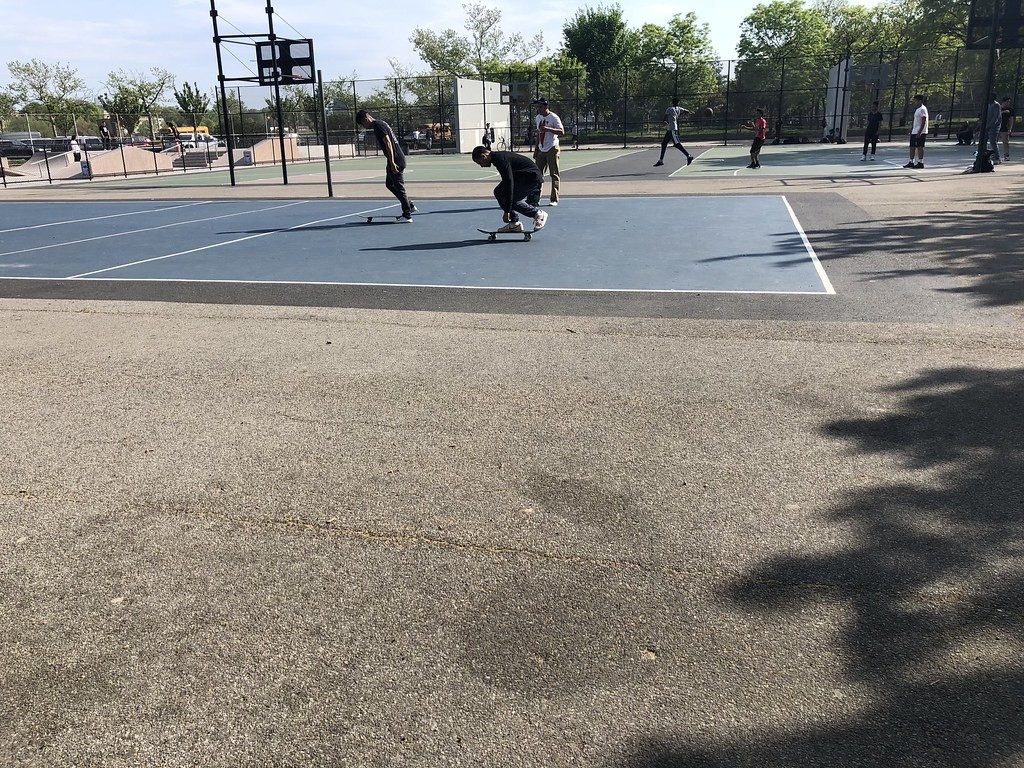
[498,221,524,232]
[549,201,558,206]
[859,154,866,161]
[912,162,924,168]
[533,209,548,231]
[410,205,417,212]
[394,216,414,223]
[870,154,875,160]
[903,161,914,167]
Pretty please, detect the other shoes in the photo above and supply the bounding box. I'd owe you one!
[956,142,963,145]
[1004,157,1010,161]
[686,156,694,165]
[653,161,664,166]
[746,162,761,168]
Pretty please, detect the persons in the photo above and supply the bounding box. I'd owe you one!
[472,146,548,232]
[903,95,928,169]
[356,110,419,223]
[970,112,983,145]
[70,135,81,162]
[527,124,537,152]
[860,99,883,161]
[425,127,432,150]
[956,120,973,146]
[848,116,869,129]
[570,121,576,132]
[933,109,942,138]
[413,128,423,150]
[484,123,491,146]
[834,127,840,142]
[533,97,564,206]
[984,91,1002,165]
[569,118,573,128]
[741,108,767,169]
[996,95,1014,161]
[653,98,695,167]
[774,118,784,140]
[166,121,184,152]
[99,121,112,151]
[572,124,578,148]
[870,132,882,143]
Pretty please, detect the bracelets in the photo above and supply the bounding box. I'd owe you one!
[1008,130,1011,132]
[880,125,883,126]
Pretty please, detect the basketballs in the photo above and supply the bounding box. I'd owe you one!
[704,108,713,117]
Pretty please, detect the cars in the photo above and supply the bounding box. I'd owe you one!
[572,116,612,142]
[512,130,538,146]
[31,133,219,154]
[0,140,46,161]
[355,129,434,150]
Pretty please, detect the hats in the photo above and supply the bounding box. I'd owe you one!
[534,97,549,105]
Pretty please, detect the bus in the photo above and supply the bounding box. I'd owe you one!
[418,122,452,142]
[157,126,210,137]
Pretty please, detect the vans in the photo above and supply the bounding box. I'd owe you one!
[0,132,42,140]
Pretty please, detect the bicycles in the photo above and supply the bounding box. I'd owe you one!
[496,136,521,152]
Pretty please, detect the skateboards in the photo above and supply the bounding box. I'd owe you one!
[476,228,545,242]
[359,215,402,224]
